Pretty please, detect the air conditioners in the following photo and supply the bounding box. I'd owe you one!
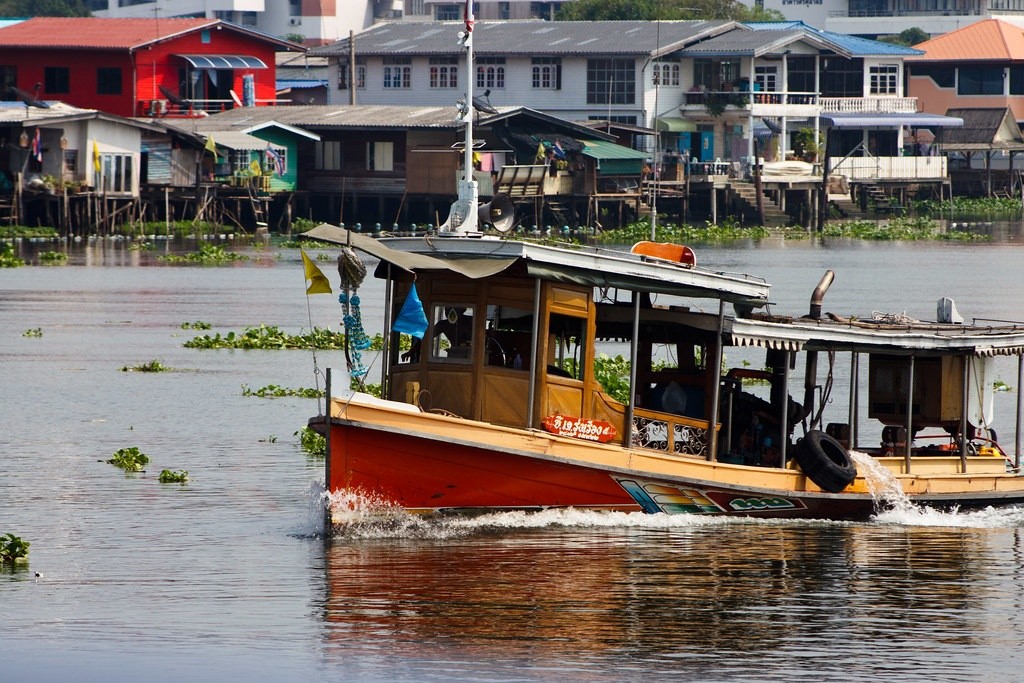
[150,100,166,113]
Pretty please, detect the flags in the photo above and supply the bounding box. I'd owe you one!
[391,283,429,342]
[250,160,261,178]
[205,136,219,164]
[536,141,565,161]
[92,142,100,172]
[266,146,284,177]
[32,128,42,162]
[301,250,332,295]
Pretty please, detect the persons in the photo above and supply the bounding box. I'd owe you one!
[401,306,473,366]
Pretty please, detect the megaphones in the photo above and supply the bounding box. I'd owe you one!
[478,192,515,232]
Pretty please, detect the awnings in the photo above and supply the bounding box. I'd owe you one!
[653,117,697,132]
[171,54,269,71]
[753,129,777,138]
[808,114,964,129]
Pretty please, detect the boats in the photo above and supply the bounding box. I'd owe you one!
[299,222,1024,538]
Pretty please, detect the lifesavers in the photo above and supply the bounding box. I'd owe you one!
[797,427,855,493]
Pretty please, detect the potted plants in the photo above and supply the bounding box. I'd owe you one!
[794,128,825,164]
